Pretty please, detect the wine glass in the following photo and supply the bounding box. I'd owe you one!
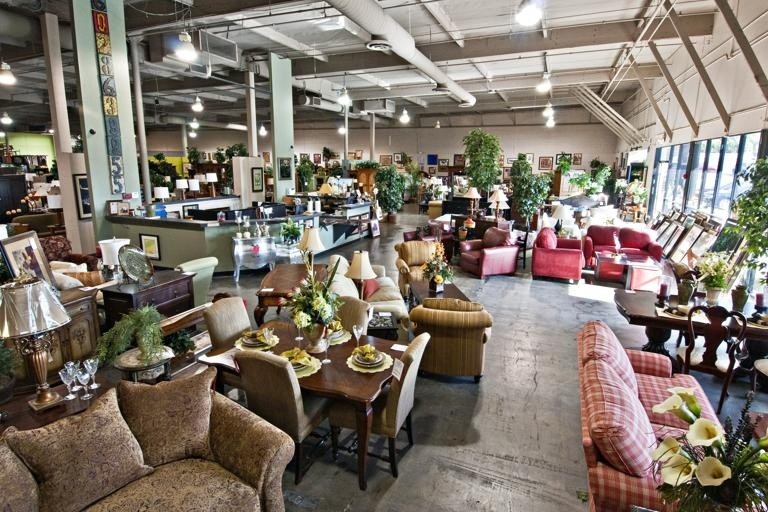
[57,358,102,402]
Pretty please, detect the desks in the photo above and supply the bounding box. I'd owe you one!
[205,319,409,488]
[404,278,472,304]
[610,288,767,377]
[1,348,197,433]
[252,261,328,326]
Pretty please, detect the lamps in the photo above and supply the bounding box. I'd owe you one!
[515,0,543,30]
[1,62,18,143]
[537,73,553,95]
[178,89,555,140]
[173,29,198,63]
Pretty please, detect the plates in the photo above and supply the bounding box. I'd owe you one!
[118,244,154,283]
[244,322,385,373]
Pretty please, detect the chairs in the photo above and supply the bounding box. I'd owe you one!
[394,238,451,300]
[124,202,736,288]
[1,215,217,348]
[411,299,494,381]
[331,332,432,479]
[329,254,409,323]
[233,351,339,484]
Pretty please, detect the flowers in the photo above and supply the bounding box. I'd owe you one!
[701,255,729,289]
[422,239,453,284]
[651,387,757,503]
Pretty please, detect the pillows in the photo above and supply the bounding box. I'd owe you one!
[582,360,658,476]
[117,366,217,462]
[581,321,639,396]
[5,387,154,512]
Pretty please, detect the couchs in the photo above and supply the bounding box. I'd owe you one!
[0,367,295,511]
[575,321,730,509]
